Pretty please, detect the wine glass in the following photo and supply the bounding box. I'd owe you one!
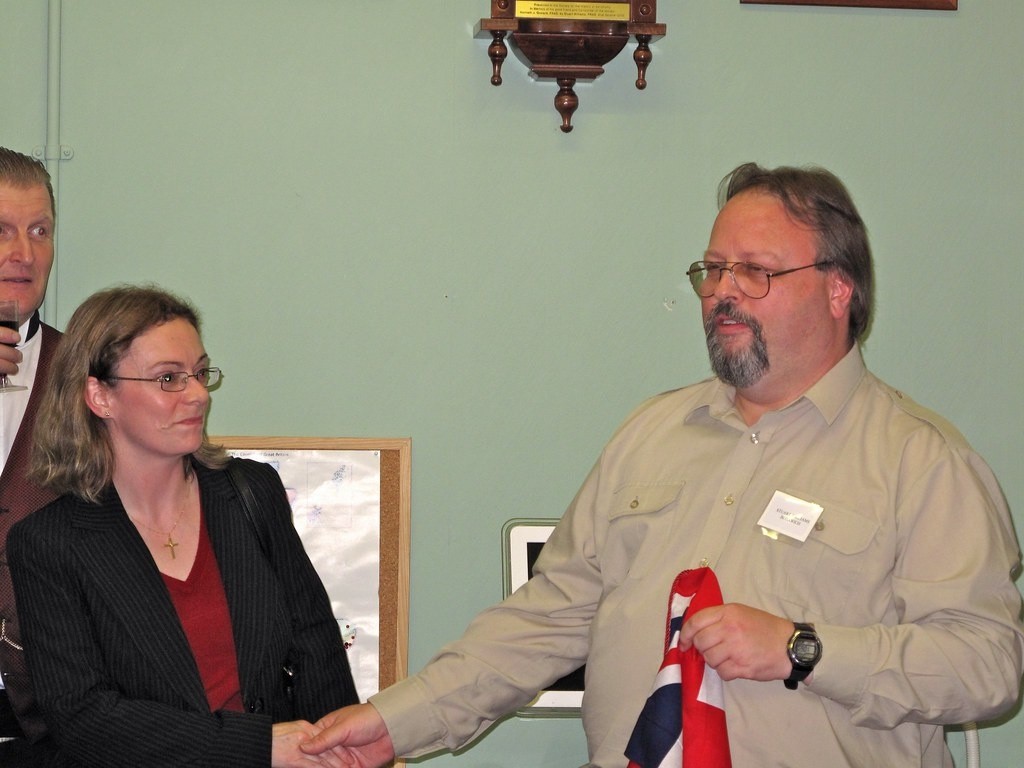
[0,296,29,393]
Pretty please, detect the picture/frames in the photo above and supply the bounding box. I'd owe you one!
[501,518,587,718]
[206,435,411,768]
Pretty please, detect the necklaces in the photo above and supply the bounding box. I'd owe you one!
[127,475,190,559]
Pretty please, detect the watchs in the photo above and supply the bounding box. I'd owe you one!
[784,621,823,690]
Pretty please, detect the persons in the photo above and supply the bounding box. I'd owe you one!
[0,145,66,767]
[5,287,360,768]
[298,160,1024,768]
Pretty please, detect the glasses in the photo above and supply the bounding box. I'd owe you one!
[98,367,223,393]
[685,258,836,300]
